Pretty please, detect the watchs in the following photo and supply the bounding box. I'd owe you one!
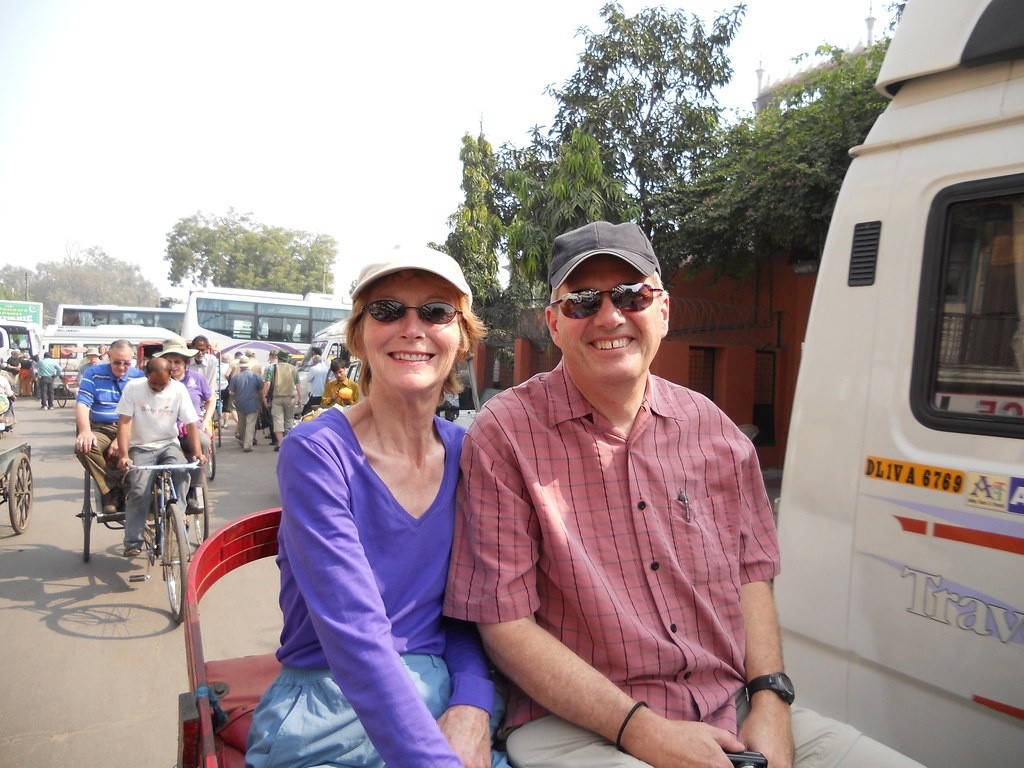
[746,672,796,705]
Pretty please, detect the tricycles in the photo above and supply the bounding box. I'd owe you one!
[56,347,88,408]
[75,423,210,625]
[137,339,223,480]
[178,505,282,768]
[0,442,33,534]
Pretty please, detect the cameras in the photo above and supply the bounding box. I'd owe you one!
[725,750,769,768]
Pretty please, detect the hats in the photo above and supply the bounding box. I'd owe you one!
[85,348,103,357]
[238,356,249,369]
[550,221,662,298]
[151,337,199,360]
[276,350,290,361]
[350,245,472,319]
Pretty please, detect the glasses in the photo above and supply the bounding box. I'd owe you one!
[550,284,665,319]
[111,359,132,367]
[362,298,466,325]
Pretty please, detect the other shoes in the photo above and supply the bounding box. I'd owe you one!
[40,405,48,411]
[104,486,122,513]
[269,441,276,445]
[186,492,205,514]
[49,406,54,410]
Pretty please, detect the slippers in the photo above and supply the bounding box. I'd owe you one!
[123,544,141,557]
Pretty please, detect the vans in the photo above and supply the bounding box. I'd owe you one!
[294,318,361,420]
[0,321,40,366]
[38,324,187,397]
[771,0,1024,768]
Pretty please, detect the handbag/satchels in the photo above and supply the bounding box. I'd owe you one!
[267,388,273,407]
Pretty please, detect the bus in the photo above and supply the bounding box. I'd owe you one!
[181,286,354,366]
[54,303,187,336]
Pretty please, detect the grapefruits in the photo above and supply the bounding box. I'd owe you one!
[339,388,352,398]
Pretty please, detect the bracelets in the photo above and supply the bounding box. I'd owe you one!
[615,701,649,749]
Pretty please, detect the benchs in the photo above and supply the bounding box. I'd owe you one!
[180,503,282,768]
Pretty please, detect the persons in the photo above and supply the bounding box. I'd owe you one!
[222,335,362,450]
[436,390,460,421]
[0,349,34,417]
[442,220,926,768]
[75,339,146,514]
[152,337,216,513]
[188,335,219,393]
[242,248,513,767]
[34,350,63,410]
[116,358,207,557]
